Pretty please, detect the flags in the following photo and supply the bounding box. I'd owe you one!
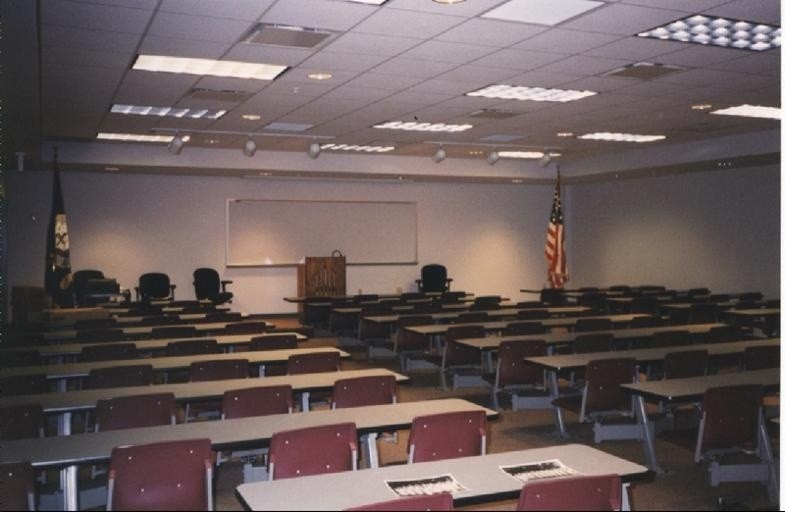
[543,165,571,289]
[44,147,74,307]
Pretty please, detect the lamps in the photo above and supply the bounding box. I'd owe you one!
[420,138,564,168]
[148,125,341,162]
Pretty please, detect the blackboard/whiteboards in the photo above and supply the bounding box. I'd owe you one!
[226,198,419,266]
